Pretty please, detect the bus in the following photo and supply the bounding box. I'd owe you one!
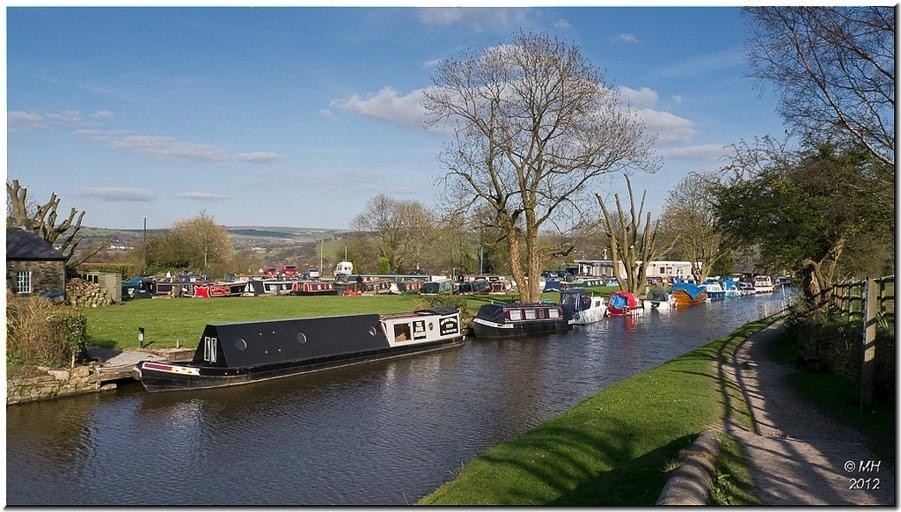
[343,277,456,296]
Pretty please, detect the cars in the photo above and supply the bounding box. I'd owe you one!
[264,265,320,281]
[122,274,191,302]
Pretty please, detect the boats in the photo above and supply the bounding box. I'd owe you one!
[132,304,470,396]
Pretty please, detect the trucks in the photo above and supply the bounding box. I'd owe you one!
[334,260,354,282]
